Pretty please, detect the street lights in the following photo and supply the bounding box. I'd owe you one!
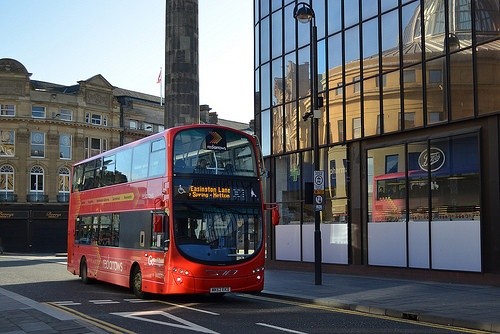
[294,1,326,290]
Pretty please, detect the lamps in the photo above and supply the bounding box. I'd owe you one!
[143,126,152,130]
[53,114,60,118]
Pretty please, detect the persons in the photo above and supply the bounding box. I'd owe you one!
[74,156,198,250]
[374,174,460,201]
[196,159,208,174]
[199,230,207,241]
[222,164,234,174]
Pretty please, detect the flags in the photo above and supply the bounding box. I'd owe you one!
[157,68,161,84]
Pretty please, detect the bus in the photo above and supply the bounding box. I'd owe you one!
[372,169,480,222]
[67,124,280,299]
